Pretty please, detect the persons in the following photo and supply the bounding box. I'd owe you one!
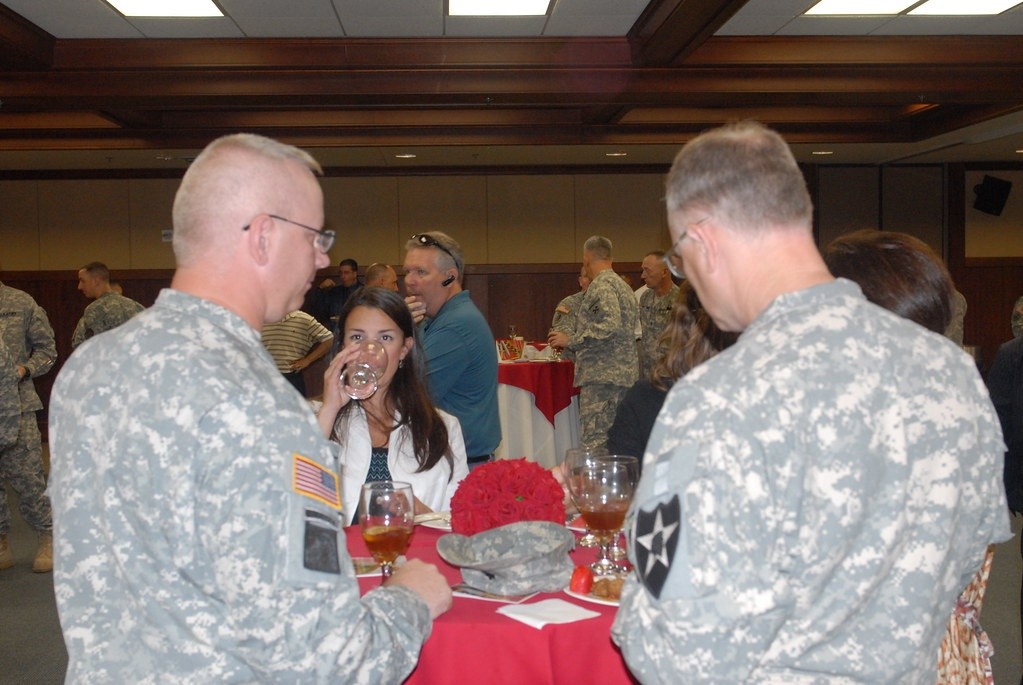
[258,309,334,402]
[313,286,472,529]
[944,289,1023,519]
[547,235,740,483]
[46,132,452,685]
[823,229,1014,685]
[610,125,1014,685]
[403,231,503,471]
[302,257,399,361]
[1,275,58,574]
[69,260,148,353]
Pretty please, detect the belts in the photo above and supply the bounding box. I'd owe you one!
[467,454,494,463]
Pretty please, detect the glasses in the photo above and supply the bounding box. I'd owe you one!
[662,218,709,279]
[410,234,459,270]
[244,215,335,254]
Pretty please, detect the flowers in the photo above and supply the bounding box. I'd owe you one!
[448,459,567,535]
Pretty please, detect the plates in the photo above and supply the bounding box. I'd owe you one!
[415,514,451,530]
[563,586,622,608]
[351,558,382,576]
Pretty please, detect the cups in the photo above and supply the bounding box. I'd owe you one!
[508,326,519,339]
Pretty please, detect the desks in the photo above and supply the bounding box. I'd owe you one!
[497,339,582,467]
[340,515,640,684]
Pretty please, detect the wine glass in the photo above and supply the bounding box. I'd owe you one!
[358,480,416,583]
[338,338,388,400]
[549,328,564,360]
[565,447,641,577]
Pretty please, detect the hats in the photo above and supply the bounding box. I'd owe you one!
[436,521,576,595]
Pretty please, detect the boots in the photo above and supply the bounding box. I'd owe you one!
[33,532,52,573]
[0,535,14,569]
